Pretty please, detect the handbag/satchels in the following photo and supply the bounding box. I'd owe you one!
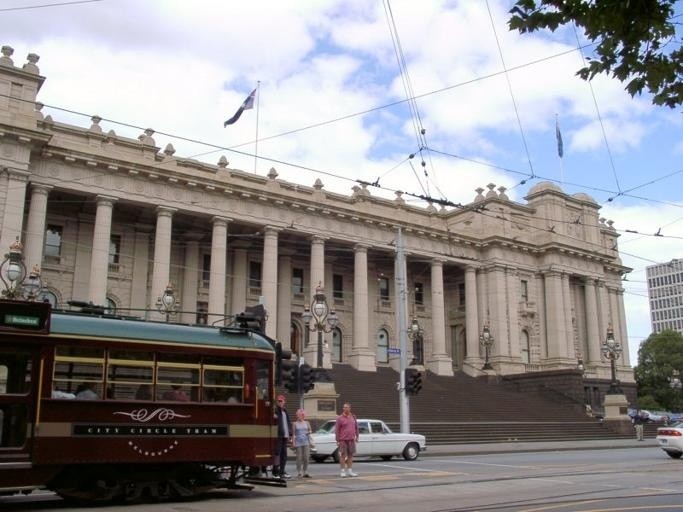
[309,438,318,453]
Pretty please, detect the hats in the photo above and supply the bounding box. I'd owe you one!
[277,395,285,401]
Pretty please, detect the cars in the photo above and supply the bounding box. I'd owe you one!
[301,419,428,463]
[625,410,683,425]
[656,420,683,459]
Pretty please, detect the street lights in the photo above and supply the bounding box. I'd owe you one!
[0,235,27,301]
[406,316,425,366]
[575,357,587,378]
[303,281,340,383]
[601,322,627,394]
[479,324,496,371]
[22,263,46,301]
[152,285,181,322]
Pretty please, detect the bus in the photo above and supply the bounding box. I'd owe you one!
[0,300,287,505]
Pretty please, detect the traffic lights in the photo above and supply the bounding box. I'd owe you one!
[301,365,316,394]
[406,368,423,394]
[286,363,299,393]
[274,342,293,384]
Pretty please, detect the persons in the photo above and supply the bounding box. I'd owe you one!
[271,394,291,479]
[289,408,312,477]
[50,376,243,406]
[334,402,357,477]
[633,408,643,441]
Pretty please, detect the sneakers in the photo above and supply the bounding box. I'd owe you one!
[272,473,292,479]
[340,471,359,477]
[297,474,311,479]
